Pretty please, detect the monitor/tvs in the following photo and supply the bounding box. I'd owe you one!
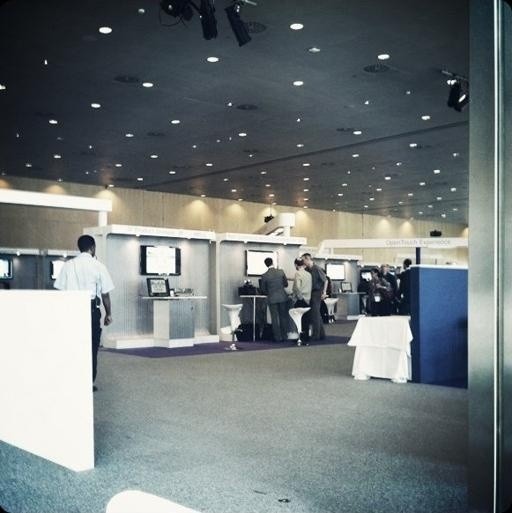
[245,250,279,276]
[50,260,65,280]
[341,282,352,292]
[0,255,13,280]
[325,263,345,281]
[140,245,180,276]
[146,278,170,297]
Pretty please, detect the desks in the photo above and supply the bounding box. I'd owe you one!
[331,292,367,315]
[240,295,293,342]
[139,295,207,339]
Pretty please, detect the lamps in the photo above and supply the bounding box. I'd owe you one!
[447,75,470,111]
[158,0,258,47]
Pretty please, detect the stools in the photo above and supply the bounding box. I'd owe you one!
[220,303,243,351]
[324,298,339,324]
[288,307,311,347]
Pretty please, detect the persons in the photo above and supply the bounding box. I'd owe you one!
[261,253,332,346]
[357,258,412,316]
[52,234,114,391]
